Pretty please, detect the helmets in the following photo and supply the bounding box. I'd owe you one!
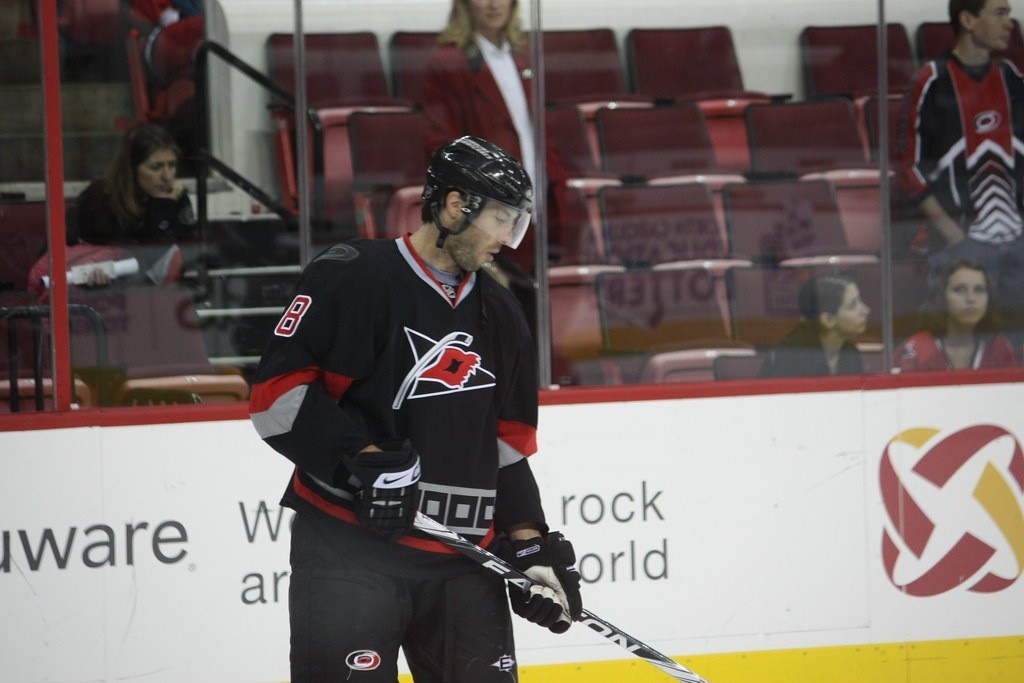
[423,135,533,214]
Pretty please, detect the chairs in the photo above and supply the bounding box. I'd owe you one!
[0,199,250,412]
[266,17,1024,388]
[60,0,197,130]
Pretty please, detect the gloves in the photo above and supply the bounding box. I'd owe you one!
[499,531,582,633]
[351,439,422,545]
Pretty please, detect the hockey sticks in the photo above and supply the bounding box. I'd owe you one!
[413,511,710,683]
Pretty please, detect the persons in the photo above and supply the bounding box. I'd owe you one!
[403,1,590,278]
[40,124,198,288]
[752,1,1024,385]
[247,132,583,683]
